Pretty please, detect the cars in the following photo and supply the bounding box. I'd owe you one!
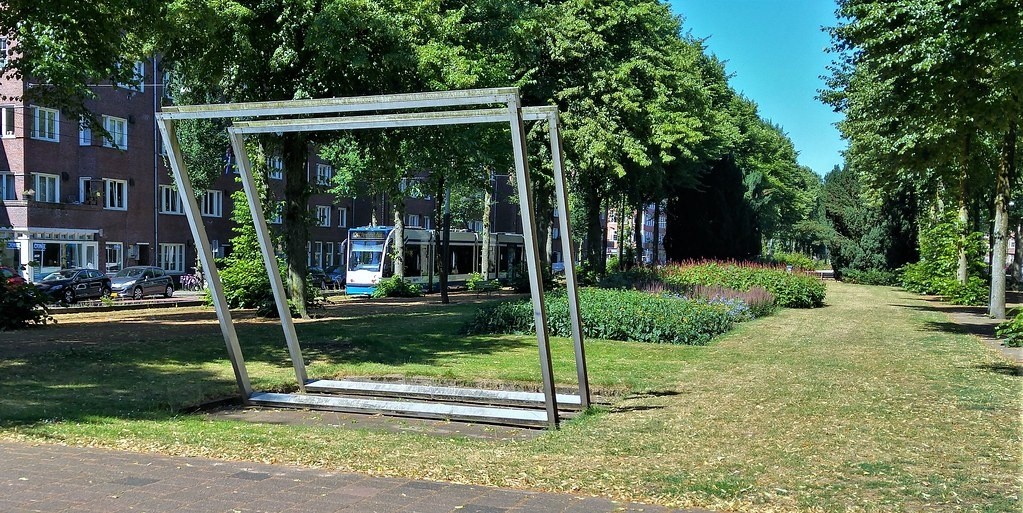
[33,268,112,304]
[0,266,27,290]
[324,264,346,289]
[110,266,174,300]
[307,265,327,290]
[552,262,565,276]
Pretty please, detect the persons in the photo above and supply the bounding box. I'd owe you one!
[62,258,67,269]
[87,260,93,268]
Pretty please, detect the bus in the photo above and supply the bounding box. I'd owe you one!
[340,226,526,295]
